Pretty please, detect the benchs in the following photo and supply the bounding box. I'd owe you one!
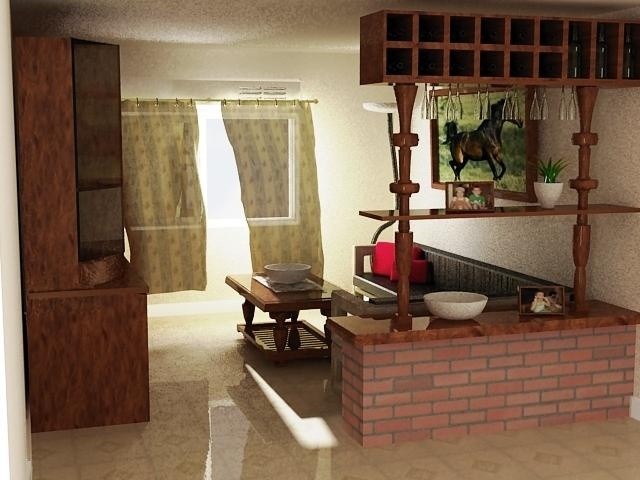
[330,242,575,319]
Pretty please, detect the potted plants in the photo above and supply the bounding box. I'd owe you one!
[533,154,566,209]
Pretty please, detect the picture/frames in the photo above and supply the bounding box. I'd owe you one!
[429,84,540,203]
[445,180,495,214]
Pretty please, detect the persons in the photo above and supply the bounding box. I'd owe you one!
[529,289,550,314]
[468,185,487,210]
[449,184,472,210]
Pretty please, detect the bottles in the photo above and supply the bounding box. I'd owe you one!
[567,22,636,79]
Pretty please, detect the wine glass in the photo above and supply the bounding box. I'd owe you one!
[418,82,578,122]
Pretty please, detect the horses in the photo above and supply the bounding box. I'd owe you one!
[441,96,524,181]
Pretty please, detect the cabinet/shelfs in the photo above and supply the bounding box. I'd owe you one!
[360,9,639,224]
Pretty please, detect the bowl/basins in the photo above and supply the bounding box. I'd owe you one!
[423,292,488,322]
[263,262,312,284]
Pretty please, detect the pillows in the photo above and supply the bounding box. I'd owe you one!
[369,241,433,283]
